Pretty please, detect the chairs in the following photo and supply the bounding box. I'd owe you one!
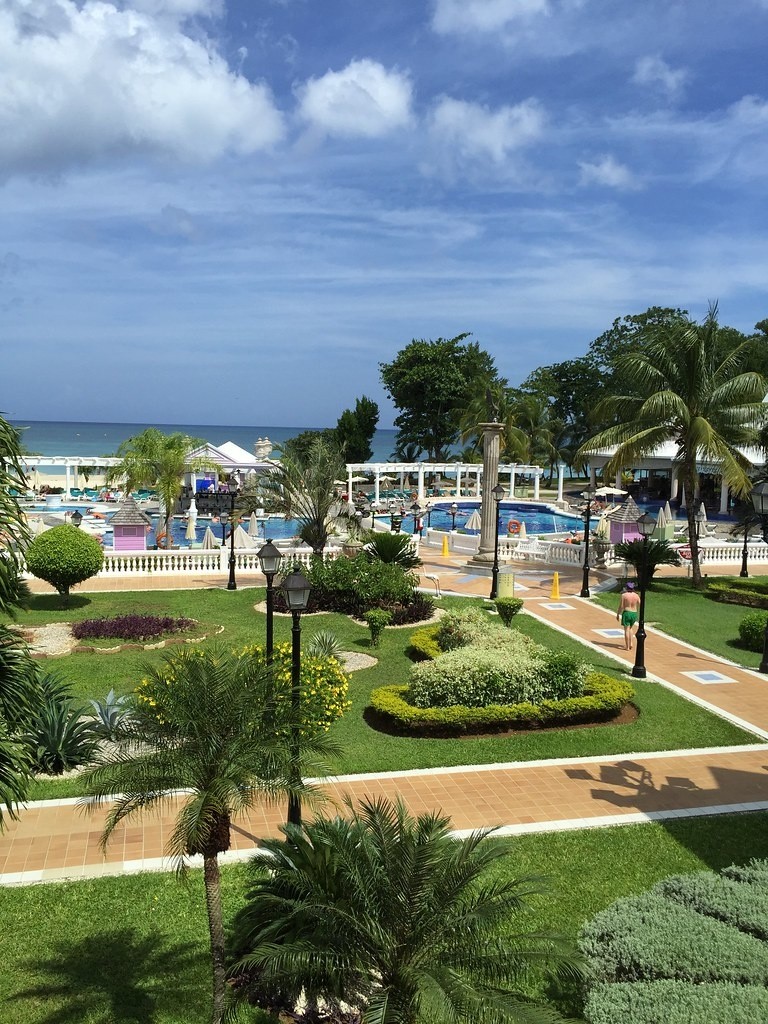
[366,489,473,502]
[70,487,157,504]
[674,524,717,538]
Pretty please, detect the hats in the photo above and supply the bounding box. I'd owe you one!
[626,582,634,590]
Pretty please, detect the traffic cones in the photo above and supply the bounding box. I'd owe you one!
[440,536,450,557]
[549,571,560,600]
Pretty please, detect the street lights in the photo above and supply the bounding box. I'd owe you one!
[631,511,657,678]
[226,475,240,590]
[580,483,596,597]
[490,485,505,600]
[276,563,316,851]
[255,538,283,781]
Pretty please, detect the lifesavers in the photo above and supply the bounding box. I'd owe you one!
[508,520,521,533]
[411,494,418,501]
[156,533,174,550]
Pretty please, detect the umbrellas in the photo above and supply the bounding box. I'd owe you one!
[664,501,672,524]
[656,508,667,542]
[580,486,628,505]
[226,525,257,549]
[346,476,369,488]
[430,481,453,486]
[35,471,41,488]
[459,477,476,483]
[405,477,410,488]
[201,526,217,550]
[36,518,45,537]
[374,474,397,481]
[700,502,708,521]
[248,511,258,539]
[518,521,527,539]
[696,522,708,535]
[464,510,482,535]
[185,517,197,540]
[596,516,607,538]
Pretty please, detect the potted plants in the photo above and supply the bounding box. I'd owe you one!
[44,485,64,506]
[343,527,364,557]
[593,530,611,552]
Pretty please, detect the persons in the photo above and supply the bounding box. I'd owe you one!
[616,583,640,650]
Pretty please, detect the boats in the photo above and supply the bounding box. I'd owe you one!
[179,525,206,530]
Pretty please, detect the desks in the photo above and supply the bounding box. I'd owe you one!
[4,533,36,552]
